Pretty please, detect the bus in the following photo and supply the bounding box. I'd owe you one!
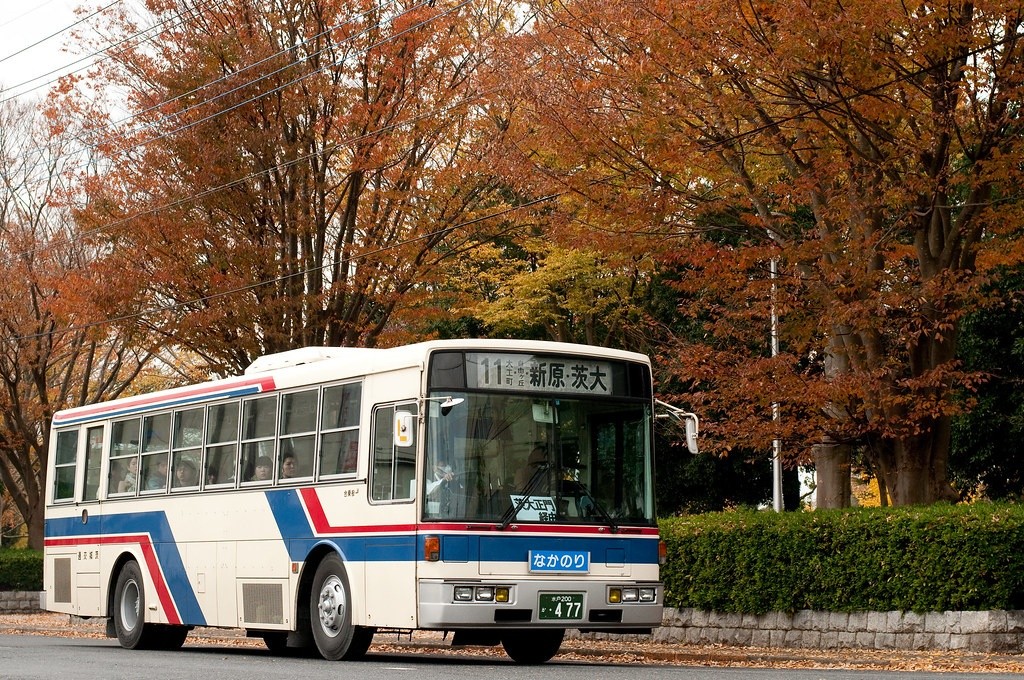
[41,338,700,663]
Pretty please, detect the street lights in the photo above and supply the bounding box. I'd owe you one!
[755,211,787,514]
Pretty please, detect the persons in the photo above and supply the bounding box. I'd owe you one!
[425,456,461,520]
[110,452,300,495]
